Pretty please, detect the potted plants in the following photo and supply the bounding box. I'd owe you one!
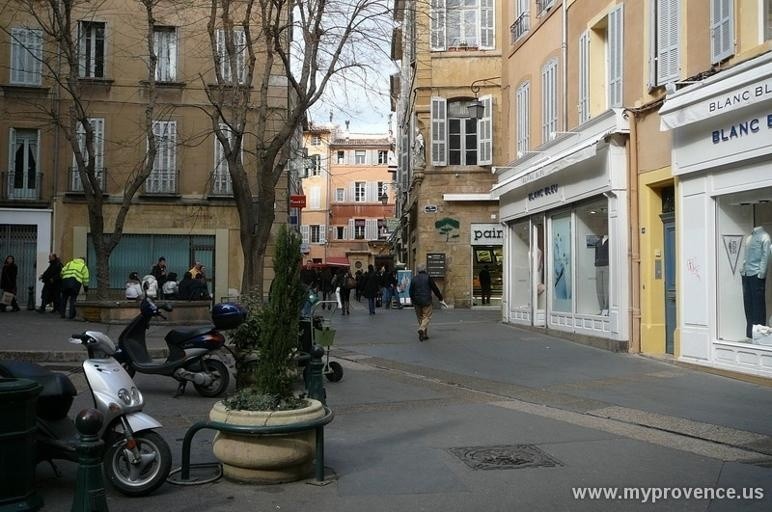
[235,283,310,397]
[208,225,327,487]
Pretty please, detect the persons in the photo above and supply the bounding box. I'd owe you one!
[125,257,212,301]
[36,253,64,314]
[409,263,448,342]
[300,259,318,318]
[741,226,771,339]
[411,128,425,168]
[1,255,21,312]
[479,265,492,305]
[59,257,90,320]
[319,264,403,316]
[528,248,542,286]
[594,234,609,316]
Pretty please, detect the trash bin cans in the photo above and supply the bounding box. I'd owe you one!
[0,378,43,510]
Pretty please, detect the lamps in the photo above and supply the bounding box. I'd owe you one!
[549,131,581,142]
[380,182,403,205]
[467,76,503,121]
[517,150,545,160]
[665,80,706,95]
[491,165,516,175]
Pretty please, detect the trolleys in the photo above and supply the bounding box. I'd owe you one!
[292,299,345,385]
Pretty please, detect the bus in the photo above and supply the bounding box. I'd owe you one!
[301,261,351,284]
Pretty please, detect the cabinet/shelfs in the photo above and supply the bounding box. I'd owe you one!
[473,247,503,296]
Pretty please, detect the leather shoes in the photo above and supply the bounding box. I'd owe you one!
[418,330,429,342]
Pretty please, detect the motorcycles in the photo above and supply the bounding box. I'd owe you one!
[114,270,240,397]
[0,329,174,496]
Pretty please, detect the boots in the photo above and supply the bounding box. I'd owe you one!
[341,302,350,316]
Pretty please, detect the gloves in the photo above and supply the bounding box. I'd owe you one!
[439,299,449,309]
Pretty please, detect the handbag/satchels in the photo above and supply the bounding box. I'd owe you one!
[0,291,14,307]
[346,273,357,290]
